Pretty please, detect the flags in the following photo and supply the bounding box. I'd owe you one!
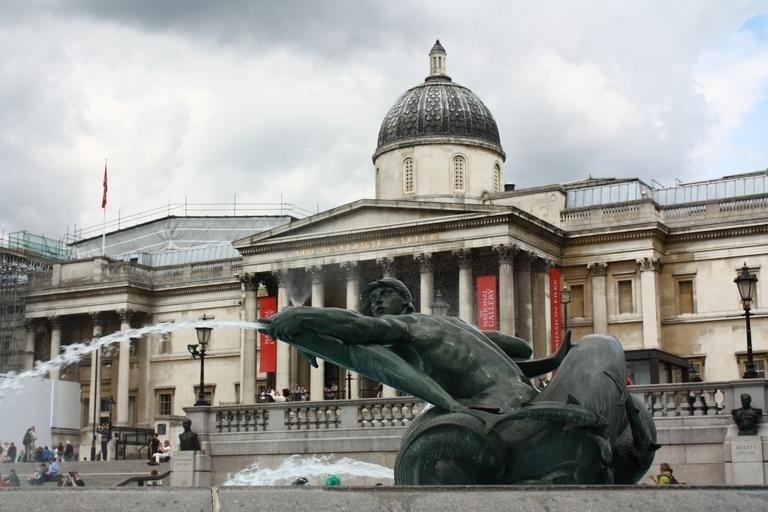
[257,296,277,372]
[476,273,497,331]
[99,159,108,211]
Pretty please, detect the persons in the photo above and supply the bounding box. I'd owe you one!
[0,424,89,488]
[153,439,171,464]
[96,422,112,462]
[258,382,340,402]
[145,470,163,486]
[730,392,762,435]
[149,432,159,461]
[626,369,633,386]
[178,419,201,450]
[270,277,574,423]
[372,382,383,398]
[649,461,679,485]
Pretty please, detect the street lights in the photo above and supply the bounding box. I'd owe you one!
[344,368,354,400]
[685,358,698,382]
[193,313,214,406]
[104,395,115,441]
[729,261,762,380]
